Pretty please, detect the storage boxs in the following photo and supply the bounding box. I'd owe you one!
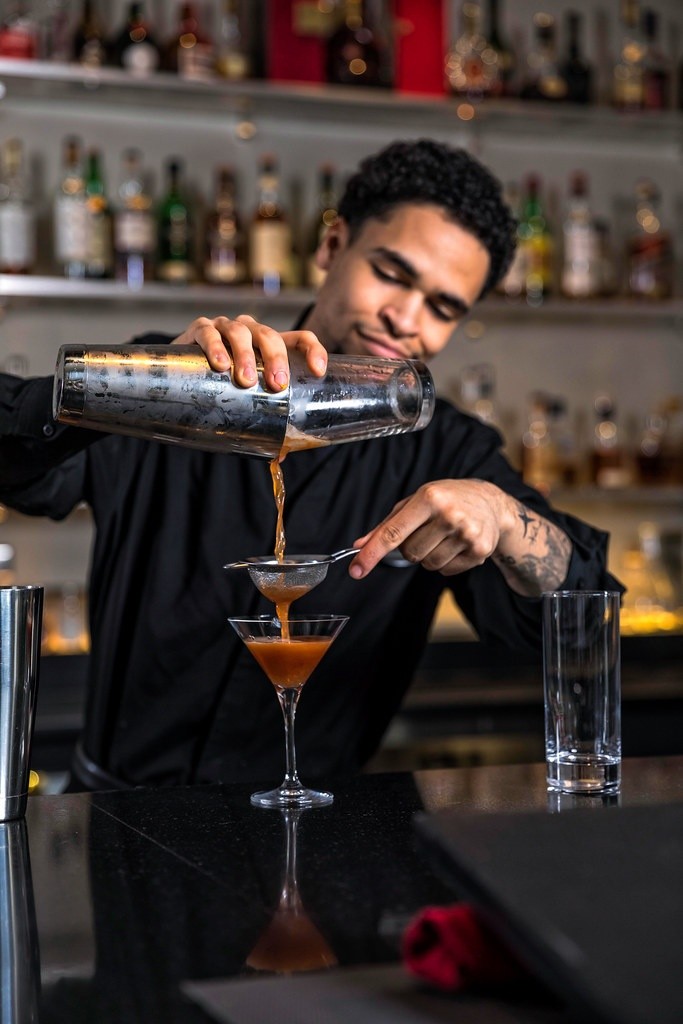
[268,0,449,102]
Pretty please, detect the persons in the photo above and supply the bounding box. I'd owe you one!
[0,137,625,795]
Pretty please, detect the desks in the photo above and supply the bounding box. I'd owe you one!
[23,754,683,1024]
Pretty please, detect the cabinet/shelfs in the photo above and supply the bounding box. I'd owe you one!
[0,56,683,512]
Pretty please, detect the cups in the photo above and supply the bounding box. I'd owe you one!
[540,591,623,796]
[49,342,289,462]
[286,355,436,455]
[0,584,44,823]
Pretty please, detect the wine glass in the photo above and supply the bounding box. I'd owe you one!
[247,801,337,967]
[227,614,351,810]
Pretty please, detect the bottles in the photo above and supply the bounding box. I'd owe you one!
[451,369,682,490]
[0,129,682,305]
[0,0,683,112]
